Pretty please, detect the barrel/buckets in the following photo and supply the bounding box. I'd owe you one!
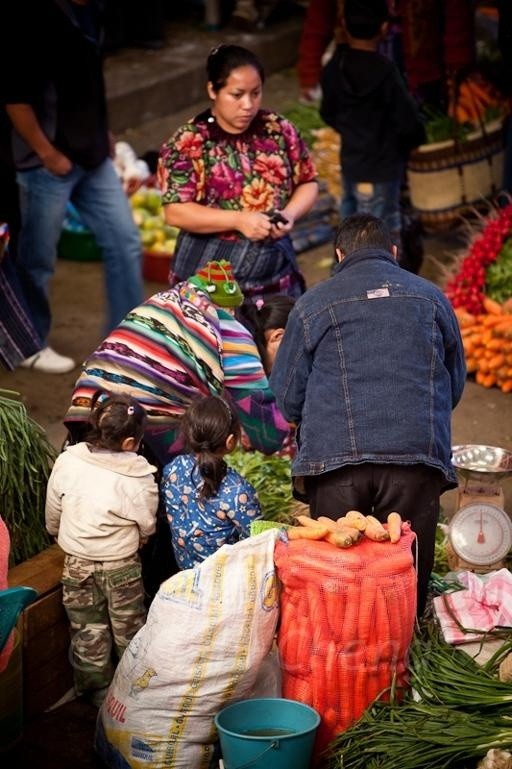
[212,700,321,768]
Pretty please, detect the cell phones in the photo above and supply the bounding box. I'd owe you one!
[269,215,289,231]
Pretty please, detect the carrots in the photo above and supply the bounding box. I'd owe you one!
[447,205,512,393]
[274,511,418,752]
[447,79,495,126]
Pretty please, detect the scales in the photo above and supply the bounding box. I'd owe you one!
[445,444,512,574]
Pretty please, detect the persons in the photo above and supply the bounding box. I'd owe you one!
[296,1,480,267]
[1,0,142,374]
[46,387,159,720]
[158,397,264,570]
[65,296,300,598]
[187,260,243,317]
[268,216,466,616]
[197,1,270,33]
[0,515,15,673]
[157,45,318,299]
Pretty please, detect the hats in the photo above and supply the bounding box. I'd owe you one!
[195,259,244,307]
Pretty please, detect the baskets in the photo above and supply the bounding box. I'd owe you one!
[402,79,504,223]
